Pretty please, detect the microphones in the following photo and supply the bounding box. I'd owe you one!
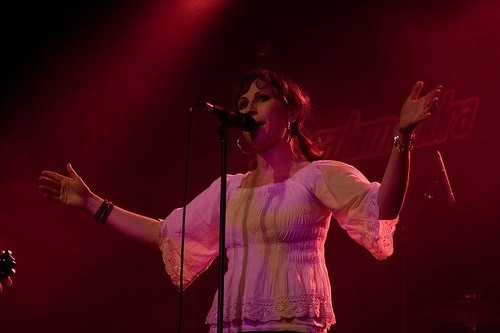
[202,101,257,131]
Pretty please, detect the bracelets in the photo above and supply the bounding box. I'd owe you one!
[393,133,415,153]
[94,198,114,222]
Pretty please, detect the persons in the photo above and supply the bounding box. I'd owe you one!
[37,68,444,333]
